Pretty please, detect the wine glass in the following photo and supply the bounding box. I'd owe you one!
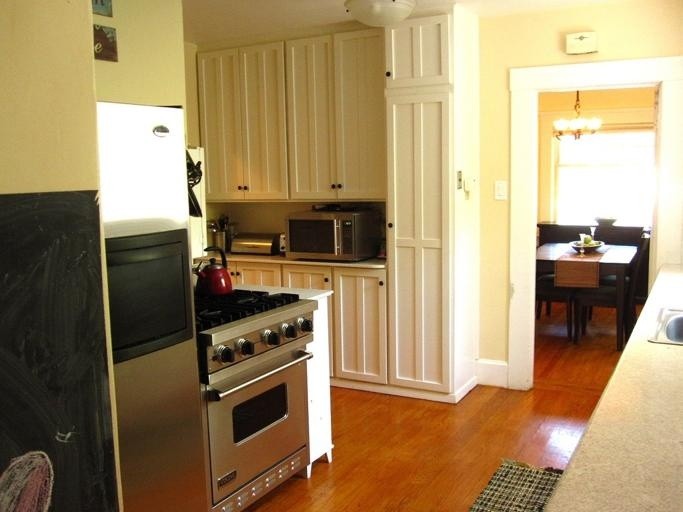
[590,226,597,241]
[578,234,586,257]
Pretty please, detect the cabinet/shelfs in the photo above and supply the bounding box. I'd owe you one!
[194,260,281,288]
[333,266,389,387]
[284,27,387,202]
[282,263,333,380]
[196,41,289,203]
[382,14,482,88]
[384,84,480,396]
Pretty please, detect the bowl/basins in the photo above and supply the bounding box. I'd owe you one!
[595,216,617,226]
[570,240,605,253]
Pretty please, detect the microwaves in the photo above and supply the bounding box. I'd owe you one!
[285,206,381,261]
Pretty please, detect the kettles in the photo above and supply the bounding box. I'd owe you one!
[196,245,233,299]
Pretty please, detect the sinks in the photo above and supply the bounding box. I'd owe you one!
[648,306,683,346]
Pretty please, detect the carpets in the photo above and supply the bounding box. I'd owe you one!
[469,455,563,512]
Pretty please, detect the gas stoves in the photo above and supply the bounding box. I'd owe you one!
[192,288,317,381]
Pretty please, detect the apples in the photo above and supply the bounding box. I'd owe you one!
[584,234,591,244]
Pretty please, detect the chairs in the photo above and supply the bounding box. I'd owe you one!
[536,223,650,352]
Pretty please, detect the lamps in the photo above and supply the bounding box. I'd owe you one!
[344,0,413,33]
[551,91,602,142]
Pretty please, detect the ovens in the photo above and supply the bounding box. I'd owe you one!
[201,333,314,512]
[103,227,194,366]
